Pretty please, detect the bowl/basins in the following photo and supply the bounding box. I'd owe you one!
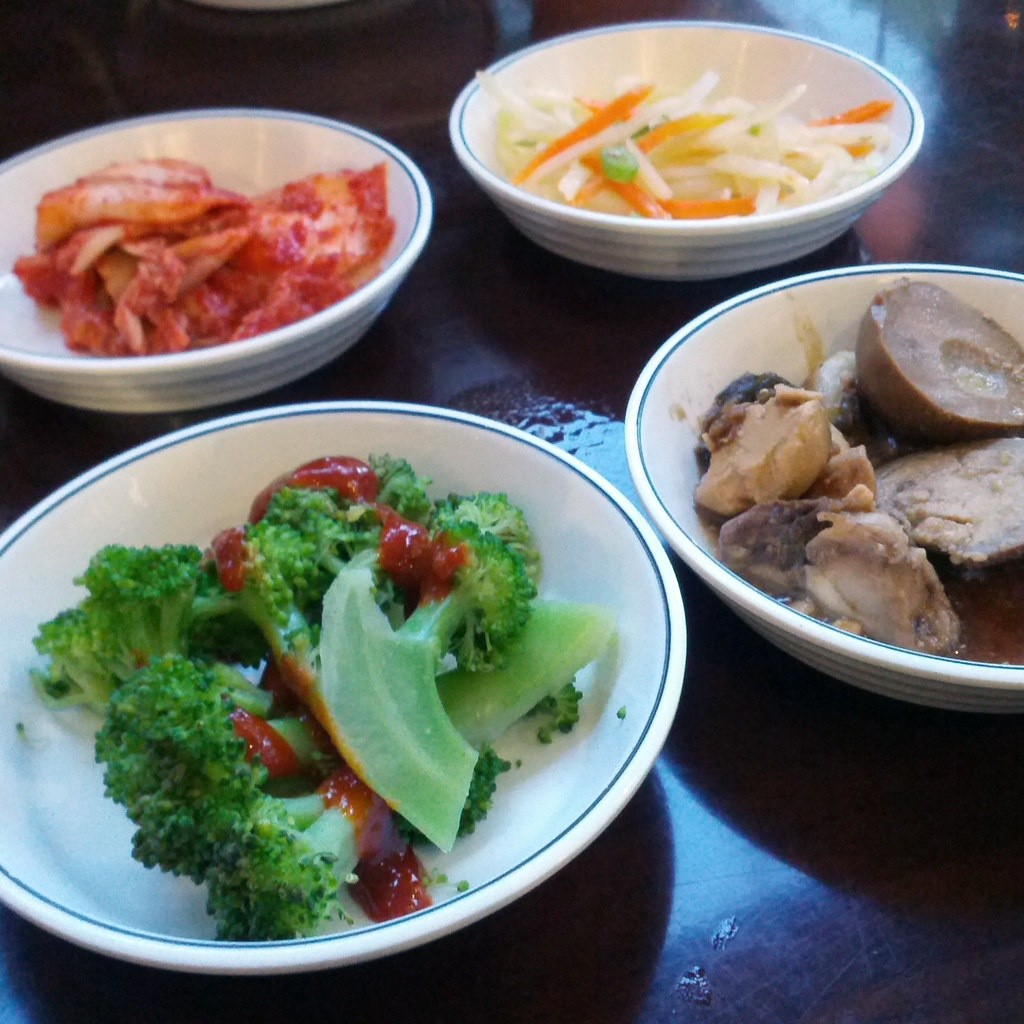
[626,262,1024,708]
[0,107,431,416]
[446,20,923,284]
[0,400,690,978]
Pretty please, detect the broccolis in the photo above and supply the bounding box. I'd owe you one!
[23,455,613,942]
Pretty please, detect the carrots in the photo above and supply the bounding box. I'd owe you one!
[513,82,892,222]
